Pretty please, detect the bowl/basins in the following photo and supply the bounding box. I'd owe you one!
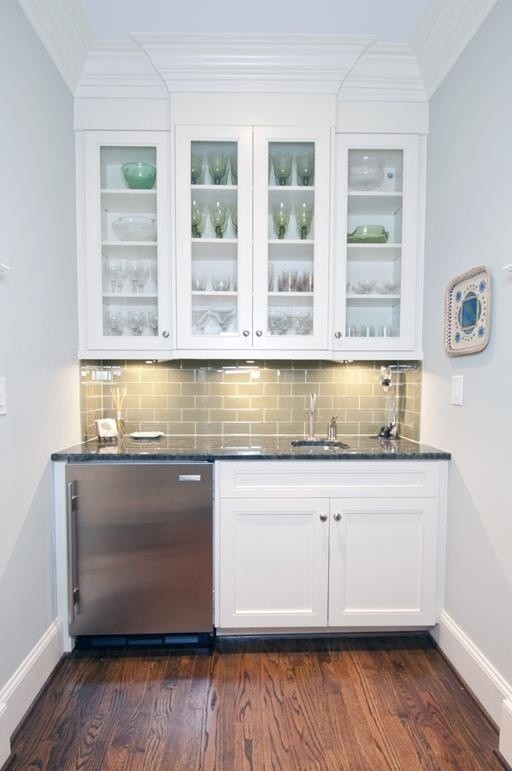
[111,215,157,242]
[120,162,154,189]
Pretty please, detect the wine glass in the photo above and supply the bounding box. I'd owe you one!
[187,150,311,242]
[102,256,157,338]
[193,309,311,336]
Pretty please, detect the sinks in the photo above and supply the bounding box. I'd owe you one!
[291,440,347,447]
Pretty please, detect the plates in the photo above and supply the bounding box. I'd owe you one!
[131,430,165,440]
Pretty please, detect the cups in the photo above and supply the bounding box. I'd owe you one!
[345,277,400,336]
[188,264,314,294]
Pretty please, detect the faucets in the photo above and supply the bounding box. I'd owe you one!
[309,393,316,438]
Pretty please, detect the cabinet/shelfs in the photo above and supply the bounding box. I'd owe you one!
[212,459,448,636]
[177,129,331,358]
[332,132,418,360]
[75,131,174,360]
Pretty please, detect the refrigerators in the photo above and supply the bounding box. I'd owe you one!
[64,460,214,637]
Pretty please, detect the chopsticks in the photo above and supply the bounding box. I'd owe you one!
[110,386,129,437]
